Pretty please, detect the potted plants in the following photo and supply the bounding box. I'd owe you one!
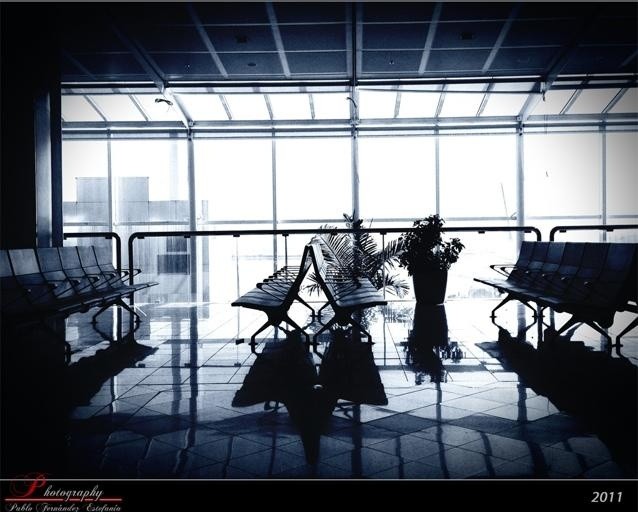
[393,211,465,306]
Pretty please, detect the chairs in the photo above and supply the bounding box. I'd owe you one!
[473,241,637,354]
[1,244,159,351]
[230,242,314,354]
[310,240,388,355]
